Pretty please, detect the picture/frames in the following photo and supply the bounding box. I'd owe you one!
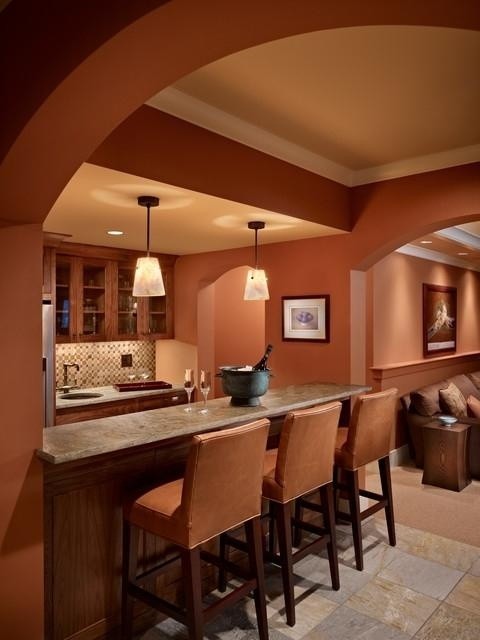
[281,294,330,344]
[422,283,457,359]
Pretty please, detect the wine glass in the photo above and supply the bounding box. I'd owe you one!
[183,368,195,413]
[126,366,151,386]
[200,368,210,413]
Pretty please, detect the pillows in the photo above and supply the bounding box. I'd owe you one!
[439,382,468,417]
[467,394,480,419]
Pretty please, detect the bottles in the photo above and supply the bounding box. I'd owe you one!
[252,345,272,370]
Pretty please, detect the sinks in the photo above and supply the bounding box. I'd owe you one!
[58,392,104,400]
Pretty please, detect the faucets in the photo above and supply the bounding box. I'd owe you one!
[56,363,80,393]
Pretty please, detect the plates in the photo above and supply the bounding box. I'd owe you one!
[440,416,457,426]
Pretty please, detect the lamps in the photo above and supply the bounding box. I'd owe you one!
[132,197,165,297]
[244,222,271,301]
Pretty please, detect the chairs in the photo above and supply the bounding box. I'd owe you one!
[121,418,270,640]
[218,402,343,627]
[293,388,399,571]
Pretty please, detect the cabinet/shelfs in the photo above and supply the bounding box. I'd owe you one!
[42,232,175,344]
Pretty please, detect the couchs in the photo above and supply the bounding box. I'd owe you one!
[400,372,480,481]
